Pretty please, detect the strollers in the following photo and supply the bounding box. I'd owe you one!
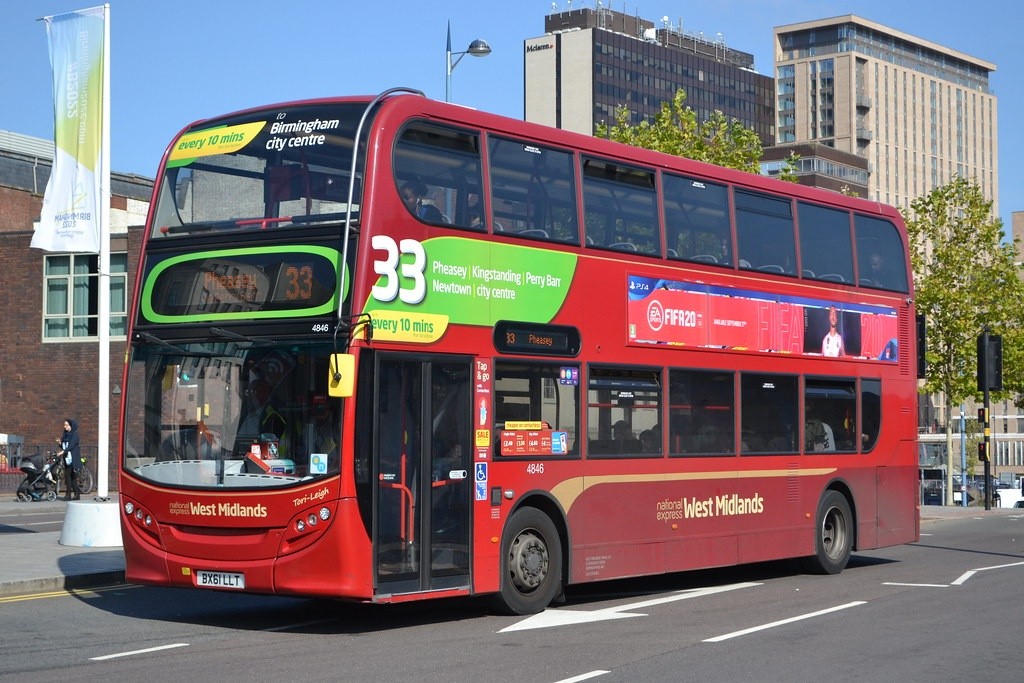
[17,453,62,502]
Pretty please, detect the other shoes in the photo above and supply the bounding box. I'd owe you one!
[71,492,81,500]
[61,491,71,501]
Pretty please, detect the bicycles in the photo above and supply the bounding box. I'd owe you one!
[50,452,94,495]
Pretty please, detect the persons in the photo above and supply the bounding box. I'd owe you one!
[820,305,846,357]
[467,207,481,228]
[56,418,81,501]
[610,400,837,454]
[237,379,286,458]
[860,253,904,292]
[400,180,442,223]
[718,234,729,265]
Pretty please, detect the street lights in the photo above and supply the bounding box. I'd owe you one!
[444,19,494,104]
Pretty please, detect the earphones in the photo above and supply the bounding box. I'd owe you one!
[417,198,419,203]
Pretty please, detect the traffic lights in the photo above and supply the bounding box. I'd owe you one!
[978,408,985,423]
[978,442,986,461]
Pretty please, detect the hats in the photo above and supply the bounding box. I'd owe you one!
[610,420,628,429]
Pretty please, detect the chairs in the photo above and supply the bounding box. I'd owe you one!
[444,206,876,286]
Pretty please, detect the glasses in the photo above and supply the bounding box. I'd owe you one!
[244,389,257,397]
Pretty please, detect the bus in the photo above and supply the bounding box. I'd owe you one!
[116,86,921,616]
